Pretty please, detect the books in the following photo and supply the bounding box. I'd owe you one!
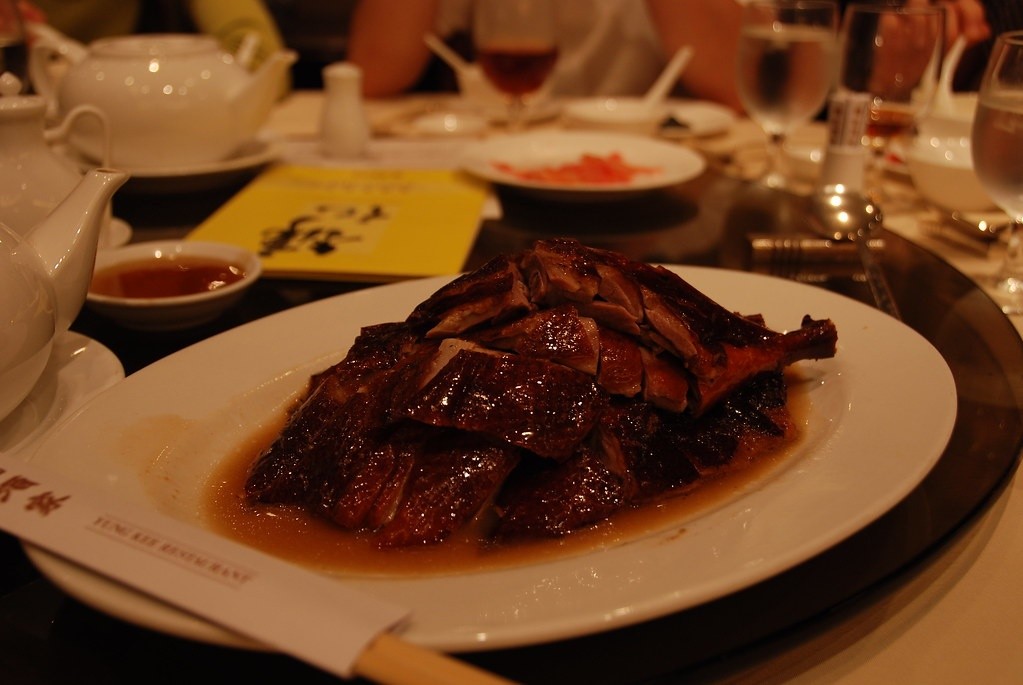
[176,132,495,280]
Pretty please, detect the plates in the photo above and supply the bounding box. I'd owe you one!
[0,331,126,457]
[81,125,289,178]
[654,97,738,140]
[20,264,958,651]
[457,129,708,193]
[559,96,667,138]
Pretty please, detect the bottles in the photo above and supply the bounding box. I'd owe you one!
[316,64,368,158]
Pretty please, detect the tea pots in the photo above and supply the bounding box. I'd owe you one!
[1,168,132,426]
[32,32,296,165]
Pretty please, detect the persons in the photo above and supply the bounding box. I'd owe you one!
[932,0,991,50]
[0,0,292,102]
[342,0,777,110]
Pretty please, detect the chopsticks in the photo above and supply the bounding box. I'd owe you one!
[352,632,518,685]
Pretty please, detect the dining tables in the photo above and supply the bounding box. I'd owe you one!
[1,89,1023,685]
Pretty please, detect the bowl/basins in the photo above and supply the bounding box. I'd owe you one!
[82,239,267,328]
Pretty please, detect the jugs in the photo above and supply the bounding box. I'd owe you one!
[0,72,115,250]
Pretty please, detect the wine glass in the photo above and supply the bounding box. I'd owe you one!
[969,33,1023,314]
[839,6,943,213]
[910,35,1017,296]
[732,0,837,193]
